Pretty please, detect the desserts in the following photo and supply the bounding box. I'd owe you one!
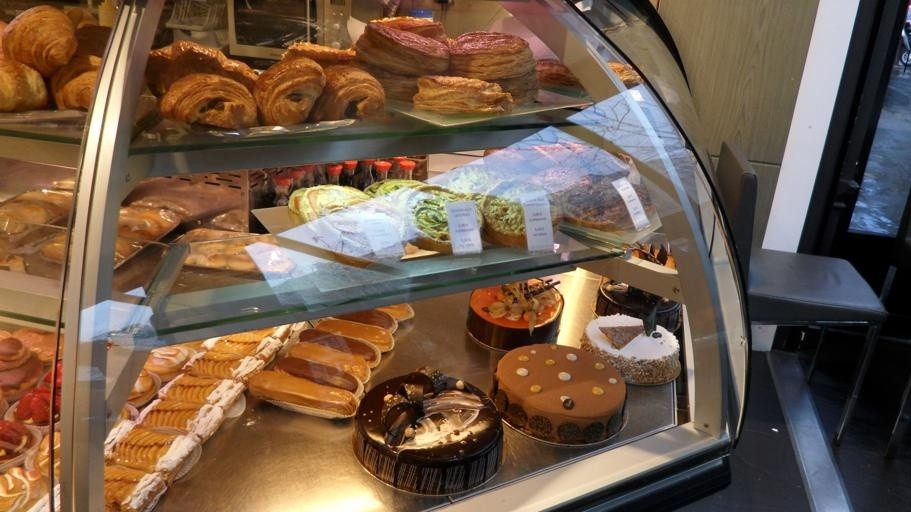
[0,303,414,512]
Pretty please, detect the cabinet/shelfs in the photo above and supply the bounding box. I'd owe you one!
[0,0,751,512]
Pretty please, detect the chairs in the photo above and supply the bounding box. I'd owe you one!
[716,137,887,448]
[806,194,909,460]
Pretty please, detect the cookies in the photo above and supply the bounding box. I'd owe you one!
[287,163,656,254]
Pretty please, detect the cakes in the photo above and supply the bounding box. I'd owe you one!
[489,343,628,445]
[354,364,503,495]
[596,242,681,332]
[466,276,565,352]
[580,307,681,384]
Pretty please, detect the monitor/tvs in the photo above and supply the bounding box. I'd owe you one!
[225,0,355,59]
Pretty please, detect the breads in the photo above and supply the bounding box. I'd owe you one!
[0,1,641,130]
[0,178,293,274]
